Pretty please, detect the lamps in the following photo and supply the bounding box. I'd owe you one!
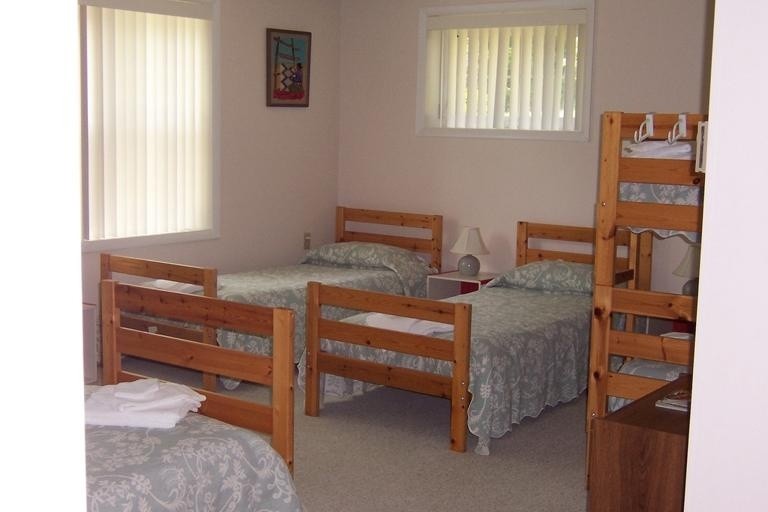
[672,246,701,296]
[449,225,490,277]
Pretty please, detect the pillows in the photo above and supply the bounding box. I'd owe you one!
[488,259,594,294]
[318,242,418,270]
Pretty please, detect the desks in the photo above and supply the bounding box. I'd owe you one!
[585,374,691,512]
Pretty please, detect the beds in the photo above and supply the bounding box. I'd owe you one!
[304,221,651,453]
[84,281,294,512]
[100,208,444,389]
[586,111,709,418]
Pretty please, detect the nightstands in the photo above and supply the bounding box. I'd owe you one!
[426,269,498,301]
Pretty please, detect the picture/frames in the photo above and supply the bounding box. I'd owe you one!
[265,27,311,107]
